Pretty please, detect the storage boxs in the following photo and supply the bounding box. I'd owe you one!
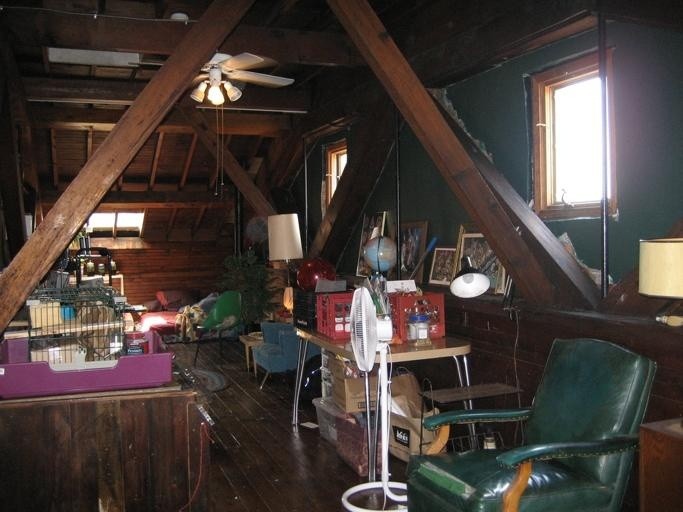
[310,347,441,479]
[290,286,354,336]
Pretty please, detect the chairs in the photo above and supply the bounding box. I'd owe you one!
[133,284,248,371]
[398,332,663,512]
[250,320,320,392]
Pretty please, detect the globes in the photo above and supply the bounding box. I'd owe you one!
[363,236,397,273]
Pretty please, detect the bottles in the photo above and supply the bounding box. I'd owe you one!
[124,325,150,353]
[408,315,432,345]
[87,258,117,273]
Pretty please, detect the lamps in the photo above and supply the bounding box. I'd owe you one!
[634,235,682,329]
[264,210,305,318]
[189,66,244,200]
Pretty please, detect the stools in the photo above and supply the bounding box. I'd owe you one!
[238,331,266,378]
[417,384,523,458]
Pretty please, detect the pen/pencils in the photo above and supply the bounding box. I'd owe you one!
[371,292,387,315]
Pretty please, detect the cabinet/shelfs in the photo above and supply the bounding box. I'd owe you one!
[631,408,682,511]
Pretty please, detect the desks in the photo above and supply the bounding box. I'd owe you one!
[288,322,483,509]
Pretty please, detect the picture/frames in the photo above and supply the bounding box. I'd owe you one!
[352,207,520,298]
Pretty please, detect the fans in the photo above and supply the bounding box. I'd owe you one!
[125,19,295,93]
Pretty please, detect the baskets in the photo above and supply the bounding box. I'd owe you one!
[316,292,356,341]
[388,291,447,342]
